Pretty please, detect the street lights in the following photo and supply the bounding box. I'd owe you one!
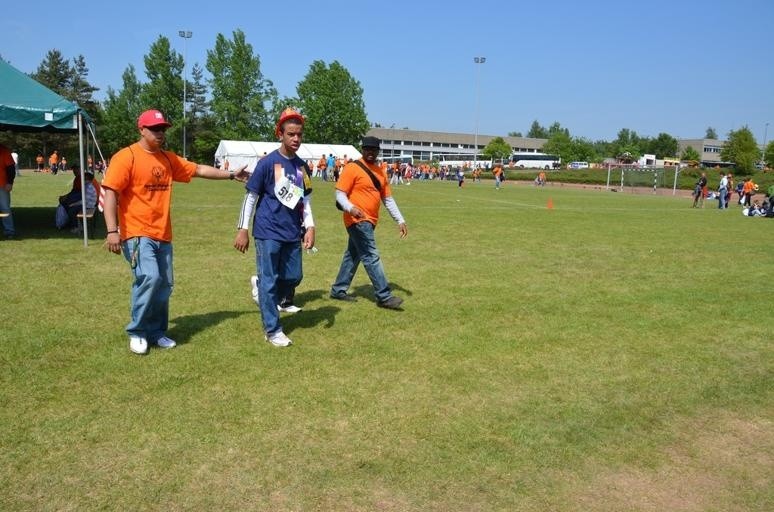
[178,30,191,158]
[761,123,769,170]
[473,57,485,173]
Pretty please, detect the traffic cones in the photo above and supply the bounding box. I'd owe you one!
[546,195,554,209]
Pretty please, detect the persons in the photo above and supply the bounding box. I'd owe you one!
[233,109,314,348]
[301,154,514,192]
[0,140,19,241]
[214,158,220,169]
[34,149,109,233]
[100,111,252,355]
[10,149,21,176]
[691,170,773,219]
[329,137,407,311]
[223,158,228,170]
[275,200,306,314]
[534,170,546,187]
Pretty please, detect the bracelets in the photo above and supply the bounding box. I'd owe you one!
[229,170,234,181]
[106,230,118,234]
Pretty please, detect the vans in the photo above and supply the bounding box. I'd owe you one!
[567,161,588,170]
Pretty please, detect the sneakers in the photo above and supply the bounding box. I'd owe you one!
[251,276,259,305]
[277,305,302,313]
[129,337,149,354]
[265,331,293,347]
[377,296,404,308]
[330,294,358,302]
[155,336,177,349]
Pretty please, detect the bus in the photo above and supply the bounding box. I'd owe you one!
[509,152,561,171]
[429,153,493,172]
[374,154,414,173]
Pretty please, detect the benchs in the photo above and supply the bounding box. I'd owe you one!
[76,207,97,238]
[0,212,11,218]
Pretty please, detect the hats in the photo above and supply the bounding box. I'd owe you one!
[362,137,380,148]
[276,107,304,137]
[138,110,172,129]
